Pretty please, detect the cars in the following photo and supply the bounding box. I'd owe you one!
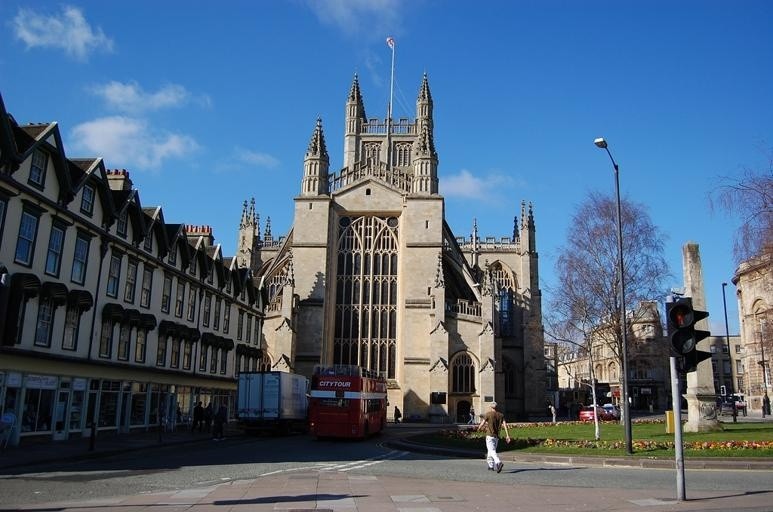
[579,403,619,421]
[725,393,747,410]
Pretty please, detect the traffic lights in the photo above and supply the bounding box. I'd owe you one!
[669,297,711,372]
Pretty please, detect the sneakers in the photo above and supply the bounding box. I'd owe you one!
[487,461,504,473]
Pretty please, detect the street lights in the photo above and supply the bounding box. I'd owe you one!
[722,282,737,422]
[594,137,632,453]
[756,331,765,418]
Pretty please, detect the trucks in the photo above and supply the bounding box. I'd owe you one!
[237,371,308,436]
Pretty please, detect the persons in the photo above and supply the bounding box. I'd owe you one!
[544,397,609,422]
[467,408,475,424]
[477,402,510,473]
[155,401,230,442]
[394,406,401,424]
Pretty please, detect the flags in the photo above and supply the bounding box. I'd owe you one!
[387,36,396,49]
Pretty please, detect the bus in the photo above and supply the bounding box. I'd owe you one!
[306,364,389,443]
